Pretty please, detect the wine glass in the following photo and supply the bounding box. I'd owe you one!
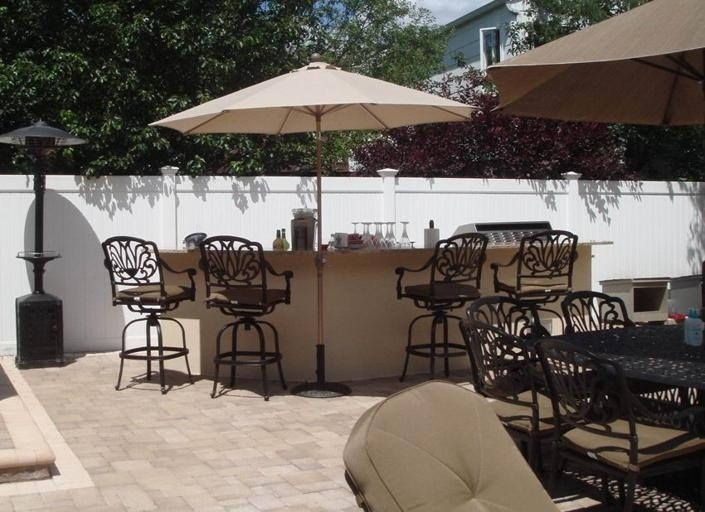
[352,222,397,249]
[400,221,412,249]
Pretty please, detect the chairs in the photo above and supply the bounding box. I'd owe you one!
[395,232,489,383]
[101,236,197,395]
[466,295,553,433]
[560,289,630,333]
[199,234,294,401]
[457,318,561,484]
[533,337,705,512]
[340,377,562,512]
[489,228,580,299]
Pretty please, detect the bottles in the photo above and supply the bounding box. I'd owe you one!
[684,304,703,346]
[281,229,289,252]
[273,230,284,252]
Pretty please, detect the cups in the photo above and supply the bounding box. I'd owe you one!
[330,233,348,248]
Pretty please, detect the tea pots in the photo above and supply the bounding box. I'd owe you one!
[290,208,318,252]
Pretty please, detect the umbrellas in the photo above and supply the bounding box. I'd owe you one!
[146,62,482,400]
[487,0,705,127]
